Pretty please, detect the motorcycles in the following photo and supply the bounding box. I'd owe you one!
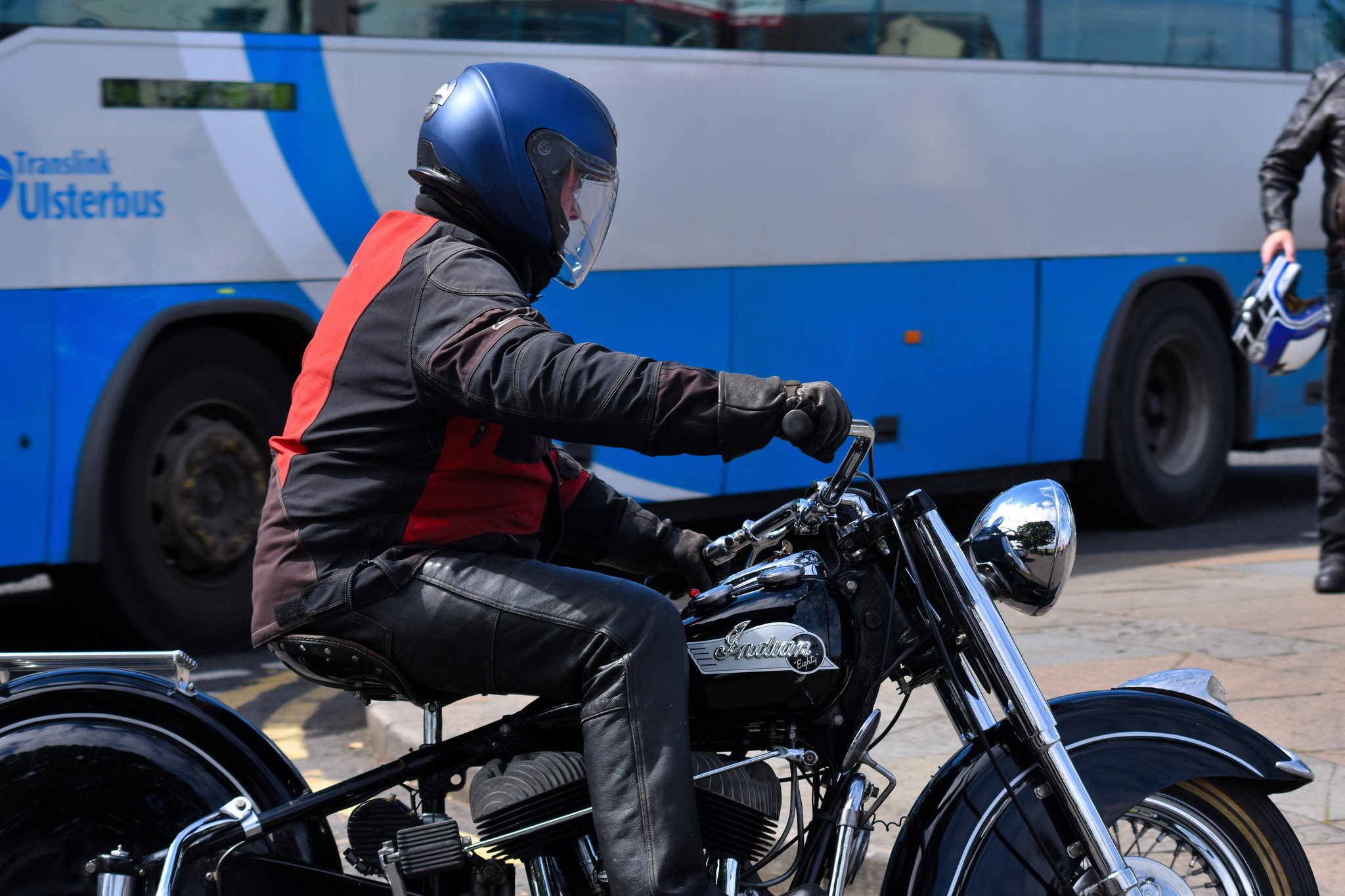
[0,408,1319,896]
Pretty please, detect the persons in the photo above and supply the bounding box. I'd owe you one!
[251,61,854,896]
[1257,60,1345,596]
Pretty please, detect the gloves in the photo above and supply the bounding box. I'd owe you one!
[592,497,733,601]
[716,371,852,464]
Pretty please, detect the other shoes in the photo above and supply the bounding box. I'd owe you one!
[1314,555,1345,591]
[779,883,827,896]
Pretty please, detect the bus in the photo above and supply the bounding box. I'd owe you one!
[0,0,1345,653]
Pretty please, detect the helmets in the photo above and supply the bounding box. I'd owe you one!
[408,63,619,290]
[1230,254,1331,375]
